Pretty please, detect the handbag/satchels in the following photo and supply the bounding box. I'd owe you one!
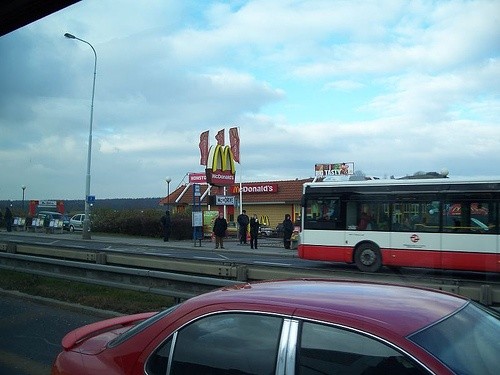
[211,234,216,240]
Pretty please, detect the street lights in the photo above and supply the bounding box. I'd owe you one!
[21,185,26,218]
[64,32,98,241]
[165,176,172,236]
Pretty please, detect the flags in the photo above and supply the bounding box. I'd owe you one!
[199,131,209,165]
[215,129,224,146]
[229,128,240,163]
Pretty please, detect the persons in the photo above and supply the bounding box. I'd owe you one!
[237,210,249,245]
[282,214,294,249]
[213,211,227,249]
[250,214,259,250]
[162,210,173,242]
[43,216,50,229]
[453,219,460,230]
[25,215,32,229]
[4,207,12,232]
[358,212,369,230]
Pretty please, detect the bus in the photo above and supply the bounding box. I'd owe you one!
[298,177,500,279]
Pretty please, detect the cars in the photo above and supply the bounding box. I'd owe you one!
[50,276,500,375]
[34,211,98,233]
[257,224,277,238]
[227,221,240,236]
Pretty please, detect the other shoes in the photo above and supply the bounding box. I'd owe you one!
[215,247,218,249]
[221,247,224,248]
